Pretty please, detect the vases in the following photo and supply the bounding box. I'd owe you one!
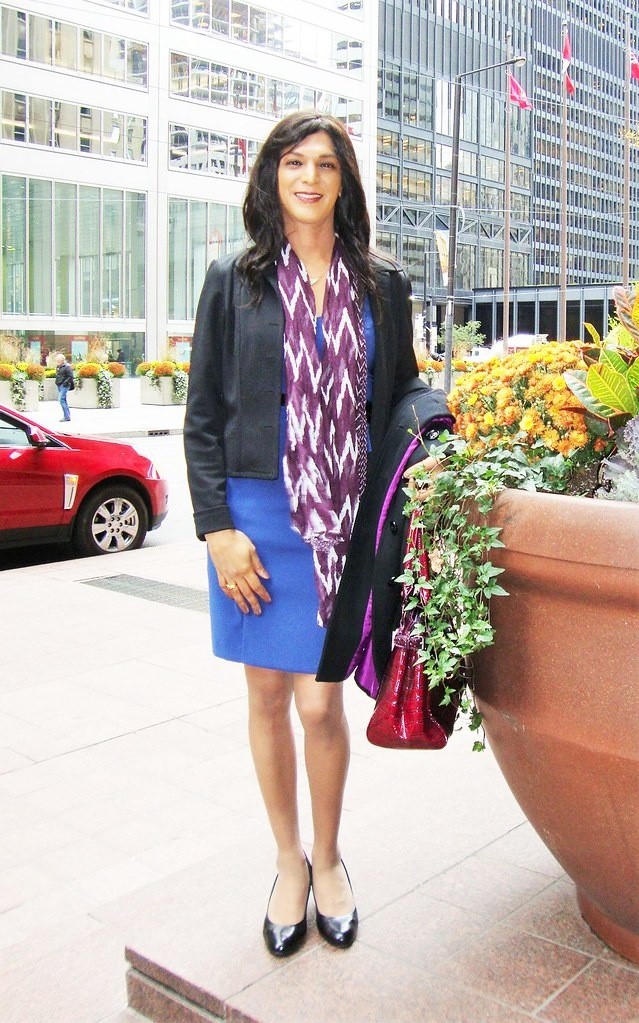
[140,375,188,405]
[66,378,121,409]
[44,377,60,402]
[0,381,39,412]
[460,487,639,965]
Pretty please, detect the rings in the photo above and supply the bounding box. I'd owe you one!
[226,584,235,590]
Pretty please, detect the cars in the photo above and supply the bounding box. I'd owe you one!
[0,403,170,559]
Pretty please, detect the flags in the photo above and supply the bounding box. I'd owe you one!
[630,53,639,80]
[562,36,574,93]
[509,74,533,110]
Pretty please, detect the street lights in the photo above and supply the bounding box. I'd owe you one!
[443,54,530,393]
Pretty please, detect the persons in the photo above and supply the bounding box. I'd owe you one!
[55,354,74,422]
[182,110,420,956]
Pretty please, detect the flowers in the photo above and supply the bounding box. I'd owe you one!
[73,362,126,410]
[135,359,190,406]
[392,283,639,753]
[44,365,57,378]
[0,361,45,412]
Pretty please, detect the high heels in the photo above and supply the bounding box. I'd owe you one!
[262,849,312,957]
[311,857,357,950]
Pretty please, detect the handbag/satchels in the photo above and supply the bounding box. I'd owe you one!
[69,380,75,391]
[367,486,467,749]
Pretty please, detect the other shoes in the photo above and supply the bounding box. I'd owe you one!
[60,417,70,422]
